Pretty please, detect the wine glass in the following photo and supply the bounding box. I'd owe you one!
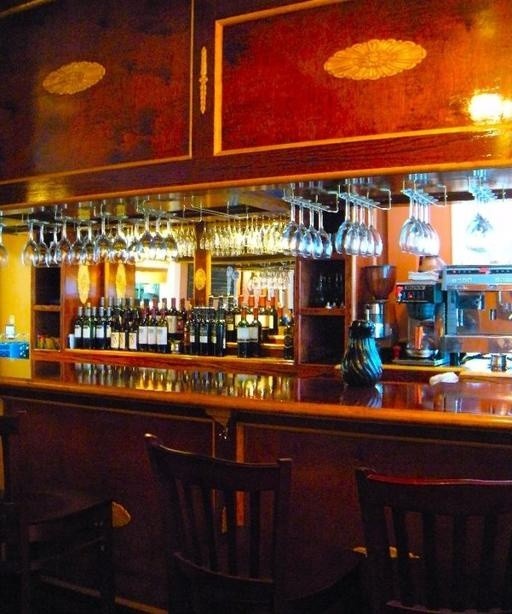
[282,195,333,258]
[334,191,383,256]
[399,188,440,257]
[466,183,498,252]
[20,208,197,268]
[0,218,7,263]
[199,218,283,259]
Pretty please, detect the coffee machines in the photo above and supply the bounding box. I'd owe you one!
[393,273,452,367]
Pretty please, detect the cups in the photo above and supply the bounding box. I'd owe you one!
[492,354,508,371]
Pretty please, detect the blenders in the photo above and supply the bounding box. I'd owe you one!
[363,264,398,341]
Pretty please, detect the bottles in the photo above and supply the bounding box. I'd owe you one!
[260,287,269,302]
[78,364,191,393]
[285,308,293,360]
[225,297,237,342]
[249,290,255,310]
[258,295,269,334]
[280,290,290,326]
[236,308,250,358]
[313,272,345,308]
[194,371,292,401]
[250,308,262,357]
[236,296,247,313]
[269,297,278,335]
[74,297,186,351]
[189,297,226,357]
[273,289,281,318]
[248,297,255,313]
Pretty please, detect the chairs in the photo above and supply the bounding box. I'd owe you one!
[0,414,115,614]
[355,466,511,614]
[144,433,365,614]
